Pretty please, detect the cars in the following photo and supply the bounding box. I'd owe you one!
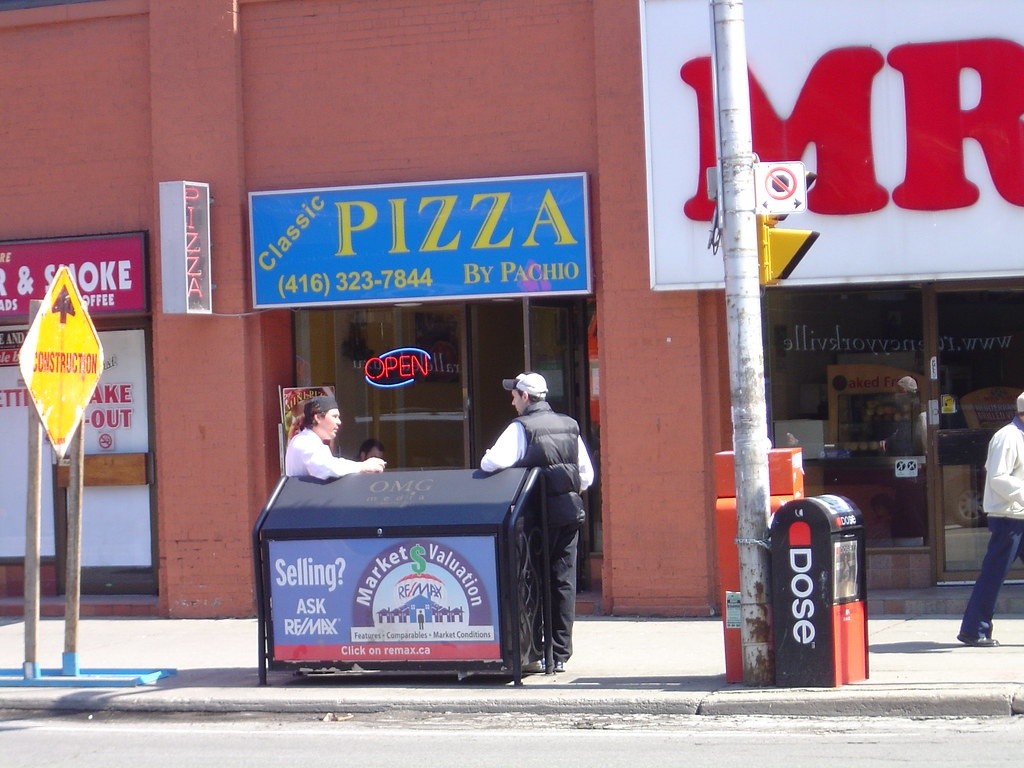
[933,424,1012,531]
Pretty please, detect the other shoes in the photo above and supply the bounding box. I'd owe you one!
[523,657,564,672]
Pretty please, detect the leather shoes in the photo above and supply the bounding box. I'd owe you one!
[956,632,1000,647]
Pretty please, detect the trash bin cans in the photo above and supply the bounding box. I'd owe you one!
[773,493,870,687]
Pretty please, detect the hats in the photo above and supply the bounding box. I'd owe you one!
[503,371,548,399]
[1016,392,1023,412]
[304,396,338,417]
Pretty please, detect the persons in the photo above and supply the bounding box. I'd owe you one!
[349,439,385,466]
[951,391,1024,648]
[283,396,386,483]
[477,372,597,676]
